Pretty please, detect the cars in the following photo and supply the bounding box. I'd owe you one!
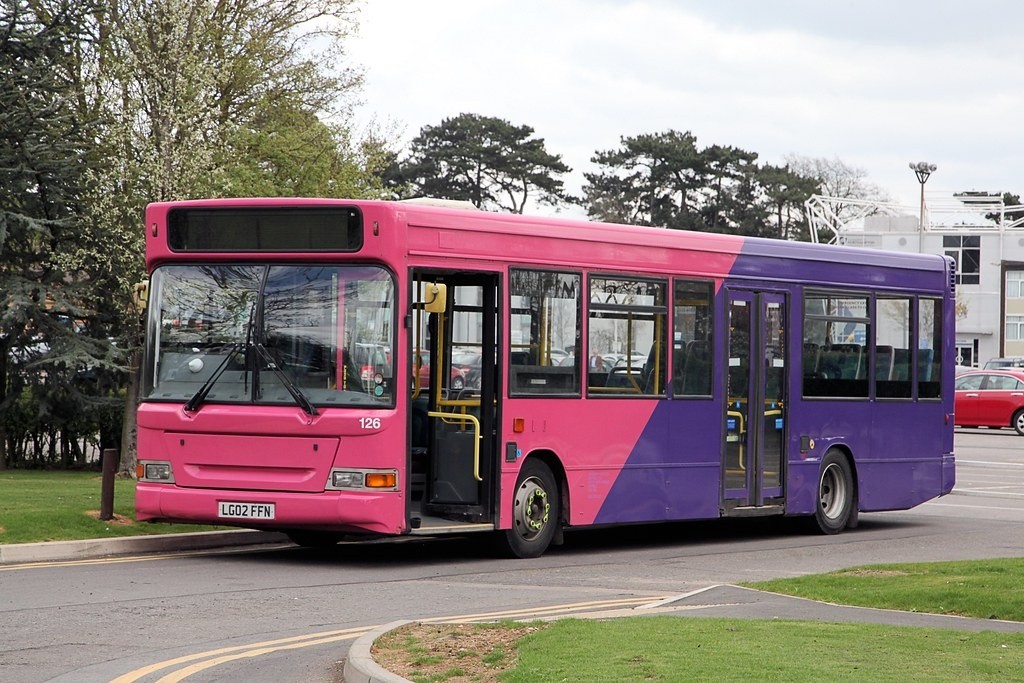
[384,349,466,388]
[956,367,1024,392]
[545,349,647,375]
[954,370,1024,435]
[452,353,483,391]
[354,343,392,396]
[984,358,1024,369]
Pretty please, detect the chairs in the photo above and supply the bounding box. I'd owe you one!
[994,380,1003,389]
[987,381,994,389]
[509,338,941,397]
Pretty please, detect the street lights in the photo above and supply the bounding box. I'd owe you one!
[909,161,937,253]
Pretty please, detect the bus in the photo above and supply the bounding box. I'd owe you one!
[135,196,957,559]
